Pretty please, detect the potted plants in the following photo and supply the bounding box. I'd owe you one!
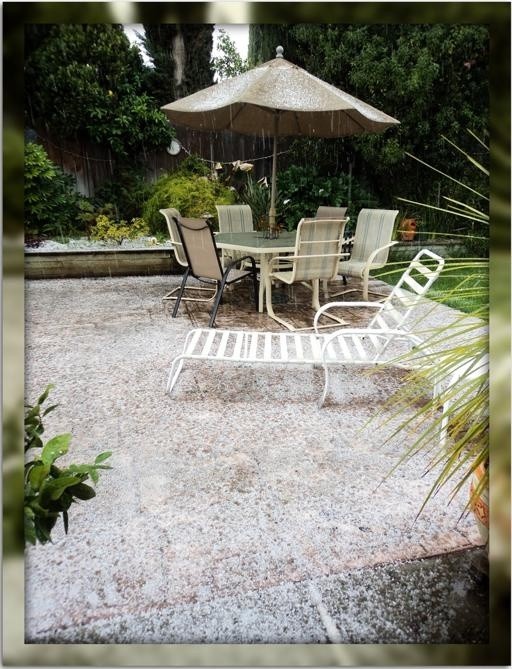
[353,129,490,544]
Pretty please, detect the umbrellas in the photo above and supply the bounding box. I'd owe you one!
[158,46,402,239]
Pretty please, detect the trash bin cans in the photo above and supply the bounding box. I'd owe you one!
[398,217,417,241]
